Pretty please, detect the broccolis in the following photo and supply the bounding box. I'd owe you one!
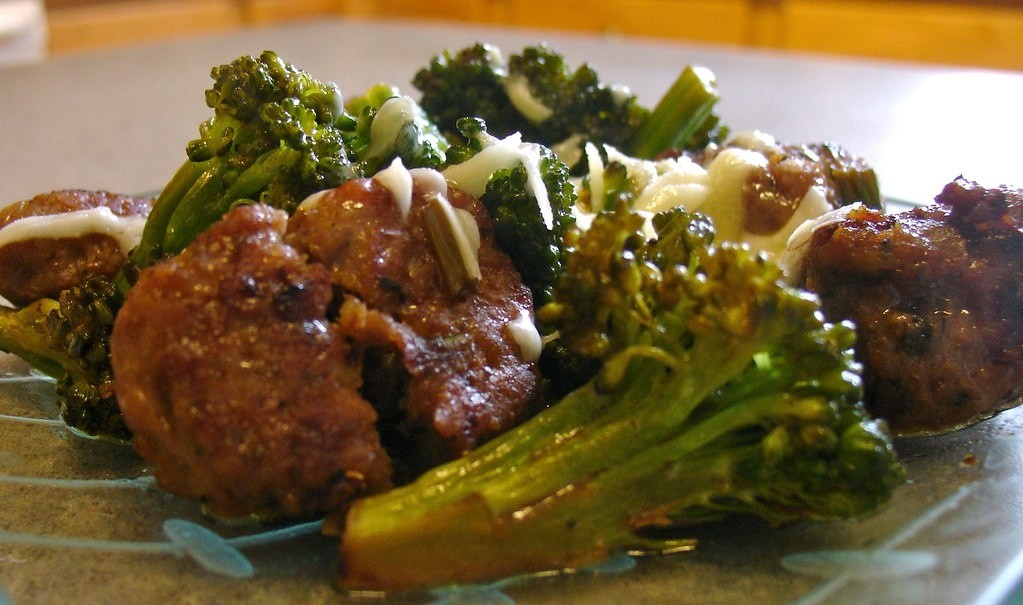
[0,43,903,591]
